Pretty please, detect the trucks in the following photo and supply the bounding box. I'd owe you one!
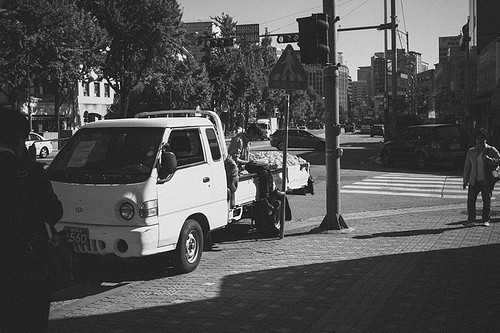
[257,117,278,141]
[46,109,315,274]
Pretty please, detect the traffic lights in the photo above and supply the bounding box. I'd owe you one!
[277,33,300,44]
[296,15,330,64]
[204,37,233,48]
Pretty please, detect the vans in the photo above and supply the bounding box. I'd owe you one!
[380,124,468,172]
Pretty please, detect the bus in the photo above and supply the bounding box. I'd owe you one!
[361,116,374,134]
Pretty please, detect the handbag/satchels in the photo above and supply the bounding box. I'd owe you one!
[31,239,74,288]
[486,147,500,182]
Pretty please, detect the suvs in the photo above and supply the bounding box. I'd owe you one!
[370,124,384,138]
[269,128,326,152]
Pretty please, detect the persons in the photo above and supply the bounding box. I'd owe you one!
[228,124,286,212]
[463,132,500,227]
[0,109,63,333]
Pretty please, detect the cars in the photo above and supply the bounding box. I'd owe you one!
[25,132,53,158]
[345,122,355,133]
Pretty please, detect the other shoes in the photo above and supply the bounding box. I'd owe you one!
[484,222,489,227]
[259,198,274,209]
[272,191,286,197]
[463,221,474,227]
